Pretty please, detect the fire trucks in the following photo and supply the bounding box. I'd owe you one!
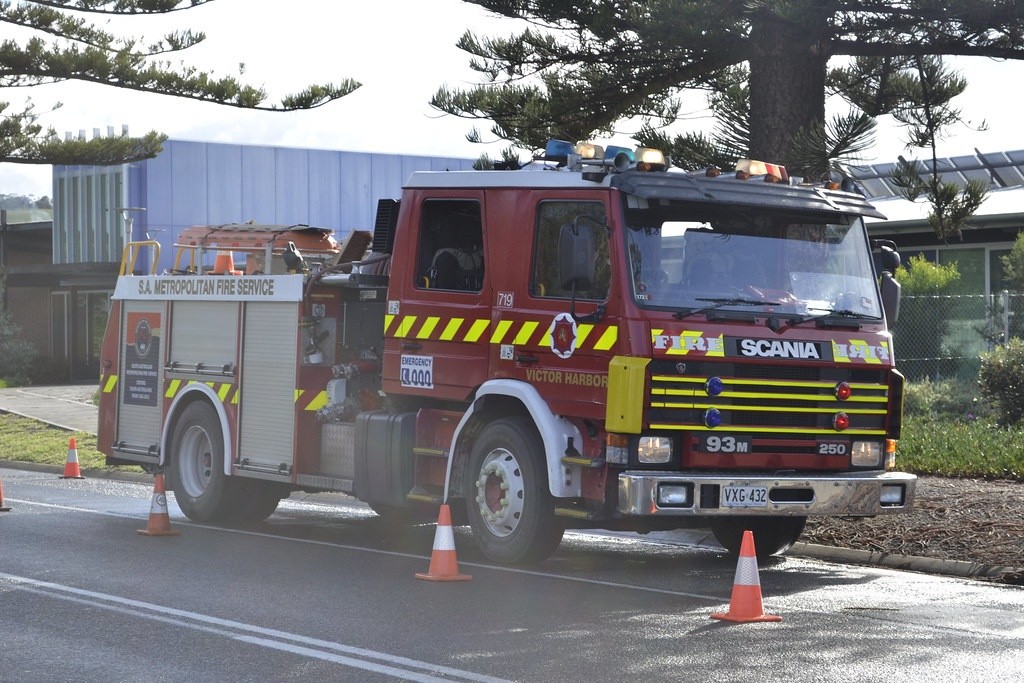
[96,137,917,568]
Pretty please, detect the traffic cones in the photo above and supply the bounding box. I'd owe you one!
[206,250,244,275]
[709,530,783,623]
[58,438,86,479]
[0,480,13,511]
[415,504,473,580]
[135,475,180,536]
